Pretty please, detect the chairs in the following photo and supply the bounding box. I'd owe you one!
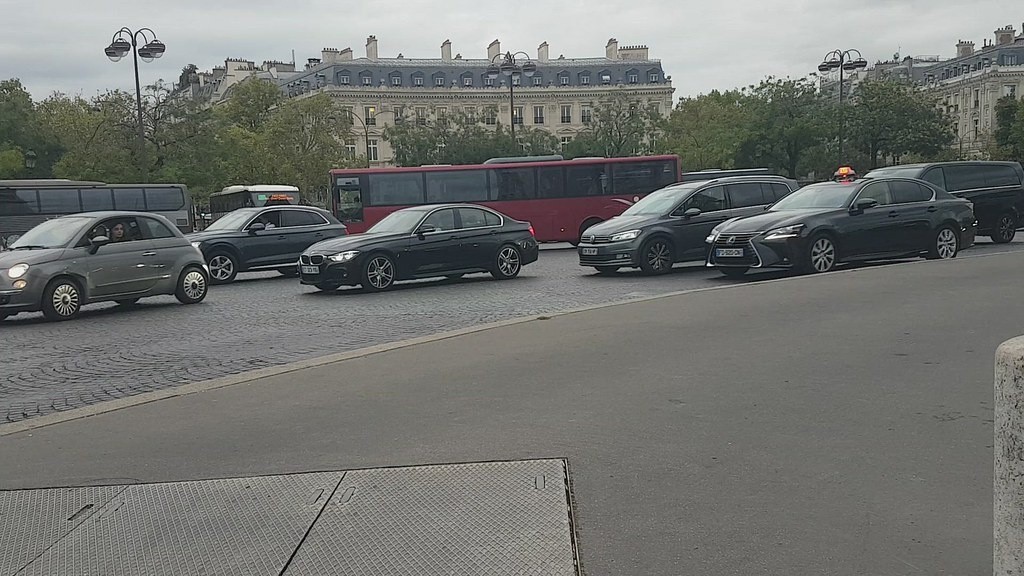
[95,226,107,237]
[439,211,453,230]
[121,222,131,241]
[870,187,889,205]
[426,215,437,224]
[260,214,269,224]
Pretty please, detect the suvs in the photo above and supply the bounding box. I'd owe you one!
[578,175,799,278]
[861,160,1024,245]
[181,205,350,285]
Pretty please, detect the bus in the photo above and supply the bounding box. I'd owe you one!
[326,154,685,249]
[209,185,301,227]
[0,179,195,253]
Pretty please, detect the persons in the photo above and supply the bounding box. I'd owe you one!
[352,195,360,208]
[259,215,274,228]
[108,222,132,241]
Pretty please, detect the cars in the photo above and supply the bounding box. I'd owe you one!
[0,210,210,322]
[295,202,541,293]
[704,175,979,275]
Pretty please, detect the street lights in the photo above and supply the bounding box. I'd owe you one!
[817,47,868,169]
[105,26,167,185]
[485,51,537,157]
[324,109,404,167]
